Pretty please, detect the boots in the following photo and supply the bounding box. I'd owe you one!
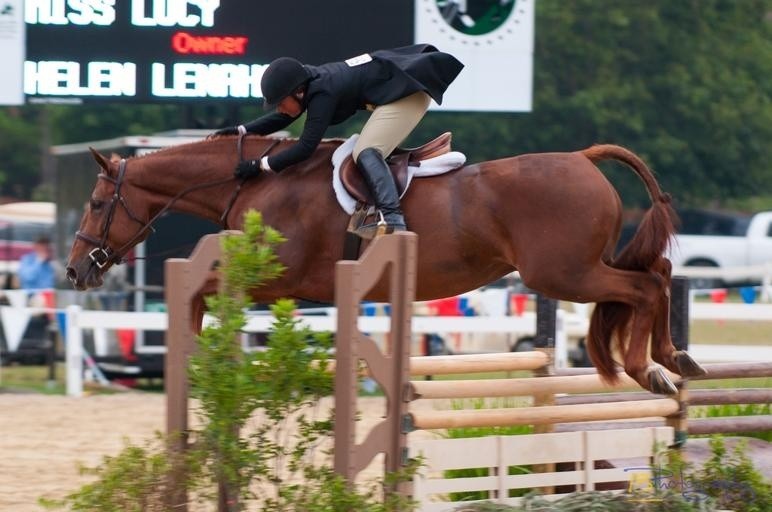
[356,148,406,239]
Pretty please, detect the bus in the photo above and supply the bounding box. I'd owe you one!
[1,195,77,367]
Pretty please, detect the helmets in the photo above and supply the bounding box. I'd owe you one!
[261,57,312,110]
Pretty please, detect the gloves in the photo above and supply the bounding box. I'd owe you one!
[207,127,239,138]
[233,160,262,178]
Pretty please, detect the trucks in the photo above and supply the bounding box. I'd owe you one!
[48,123,537,391]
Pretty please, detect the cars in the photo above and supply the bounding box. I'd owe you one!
[664,206,772,296]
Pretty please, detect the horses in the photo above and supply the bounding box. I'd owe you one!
[64,134,710,396]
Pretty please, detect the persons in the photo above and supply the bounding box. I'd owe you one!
[18,241,56,288]
[207,43,468,243]
